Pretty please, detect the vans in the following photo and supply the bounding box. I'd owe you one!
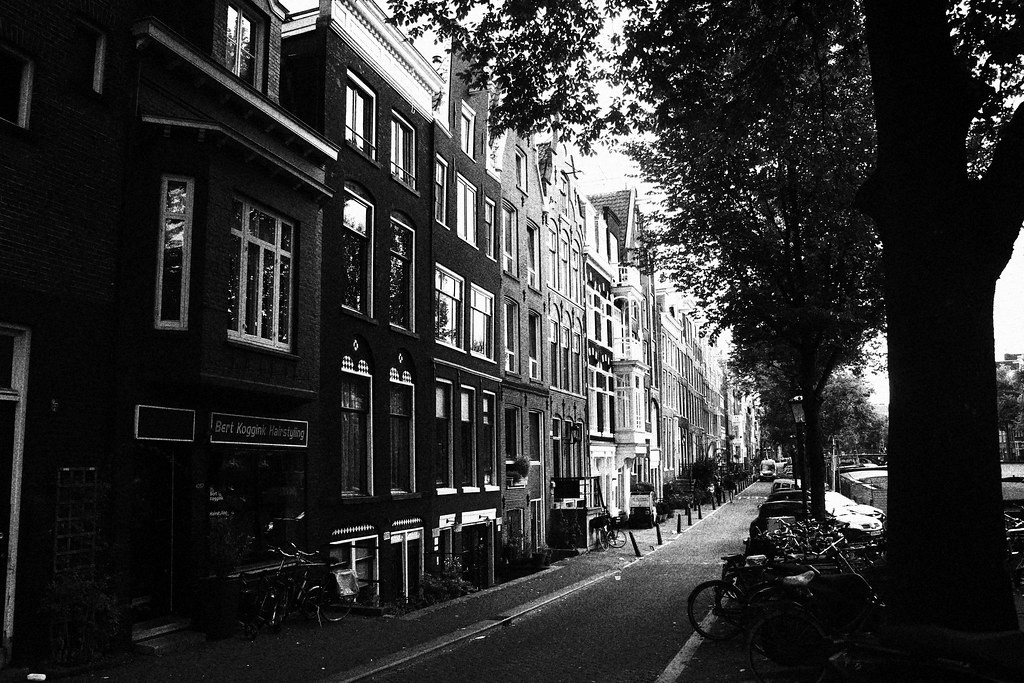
[760,460,778,481]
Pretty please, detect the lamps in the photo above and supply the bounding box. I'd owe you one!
[564,424,578,445]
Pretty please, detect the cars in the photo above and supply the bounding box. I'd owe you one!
[767,479,886,524]
[749,501,887,552]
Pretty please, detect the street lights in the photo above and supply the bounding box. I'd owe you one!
[720,382,731,483]
[789,396,807,516]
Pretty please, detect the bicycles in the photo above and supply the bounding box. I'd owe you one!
[242,540,357,642]
[687,514,1024,683]
[597,507,627,552]
